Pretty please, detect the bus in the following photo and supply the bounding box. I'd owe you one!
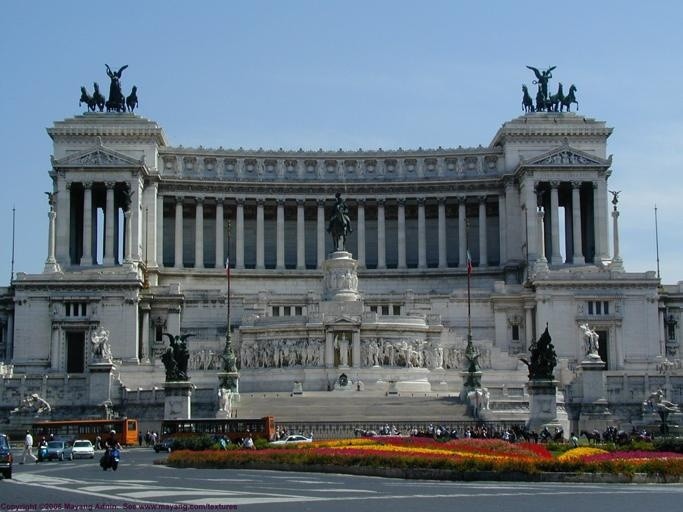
[28,419,138,448]
[159,415,276,444]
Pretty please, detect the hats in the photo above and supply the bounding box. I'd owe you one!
[109,429,116,435]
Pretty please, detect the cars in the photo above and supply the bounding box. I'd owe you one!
[0,432,15,480]
[71,439,95,459]
[267,433,312,446]
[154,439,174,453]
[37,439,74,462]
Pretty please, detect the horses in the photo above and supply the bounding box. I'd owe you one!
[520,429,539,443]
[580,430,598,445]
[353,428,377,436]
[560,84,578,112]
[331,204,348,252]
[522,84,532,113]
[92,82,105,112]
[126,85,138,112]
[79,86,96,111]
[549,82,565,112]
[536,85,546,112]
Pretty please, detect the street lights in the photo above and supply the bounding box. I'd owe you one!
[651,353,673,402]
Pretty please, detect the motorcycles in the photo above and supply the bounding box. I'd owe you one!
[37,444,48,463]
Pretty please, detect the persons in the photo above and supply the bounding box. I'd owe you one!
[379,423,647,448]
[275,425,287,440]
[18,430,55,464]
[666,315,677,340]
[106,430,123,456]
[96,434,101,450]
[537,70,552,99]
[13,394,52,414]
[219,435,227,450]
[326,197,353,234]
[137,429,159,446]
[111,71,119,81]
[90,326,110,359]
[646,390,680,413]
[243,433,255,449]
